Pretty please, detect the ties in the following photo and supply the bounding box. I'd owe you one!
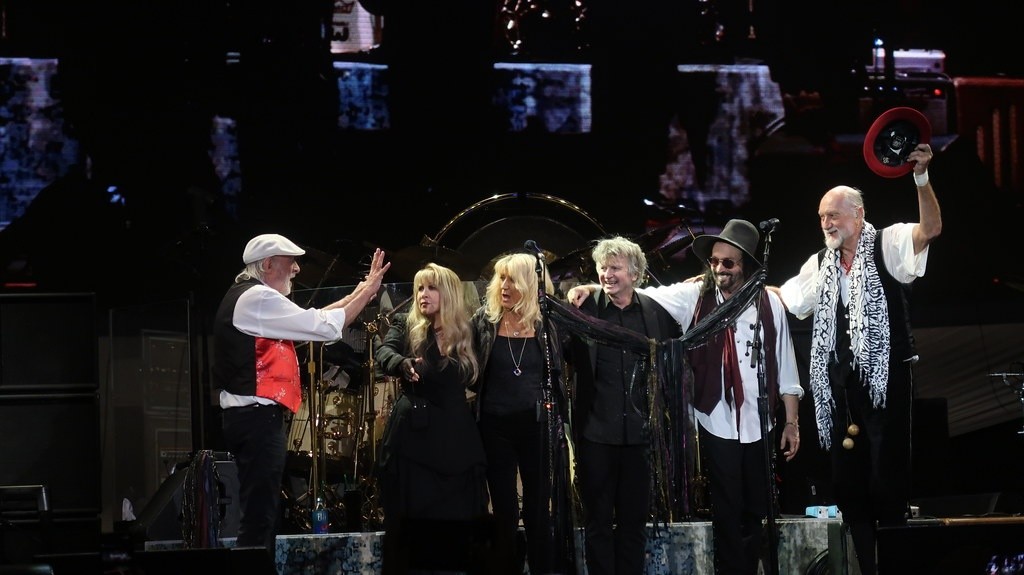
[722,325,744,430]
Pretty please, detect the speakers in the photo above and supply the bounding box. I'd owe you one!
[128,461,240,542]
[877,521,1024,575]
[0,289,103,575]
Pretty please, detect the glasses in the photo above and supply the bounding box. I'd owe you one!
[706,256,745,269]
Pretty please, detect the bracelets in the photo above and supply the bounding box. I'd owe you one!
[785,423,800,428]
[913,167,929,186]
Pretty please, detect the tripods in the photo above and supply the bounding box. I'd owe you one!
[281,342,352,536]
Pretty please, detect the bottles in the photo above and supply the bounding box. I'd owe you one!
[312,497,328,534]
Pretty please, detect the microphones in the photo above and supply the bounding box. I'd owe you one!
[759,218,780,231]
[523,240,545,260]
[988,373,1024,377]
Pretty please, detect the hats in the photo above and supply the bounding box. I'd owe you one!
[242,233,306,264]
[863,107,931,178]
[692,219,763,270]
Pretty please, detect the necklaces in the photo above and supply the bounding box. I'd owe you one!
[841,254,855,275]
[505,312,527,376]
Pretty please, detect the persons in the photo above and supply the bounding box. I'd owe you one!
[375,262,489,517]
[685,145,942,520]
[563,236,682,575]
[468,252,563,575]
[212,233,391,549]
[567,219,804,575]
